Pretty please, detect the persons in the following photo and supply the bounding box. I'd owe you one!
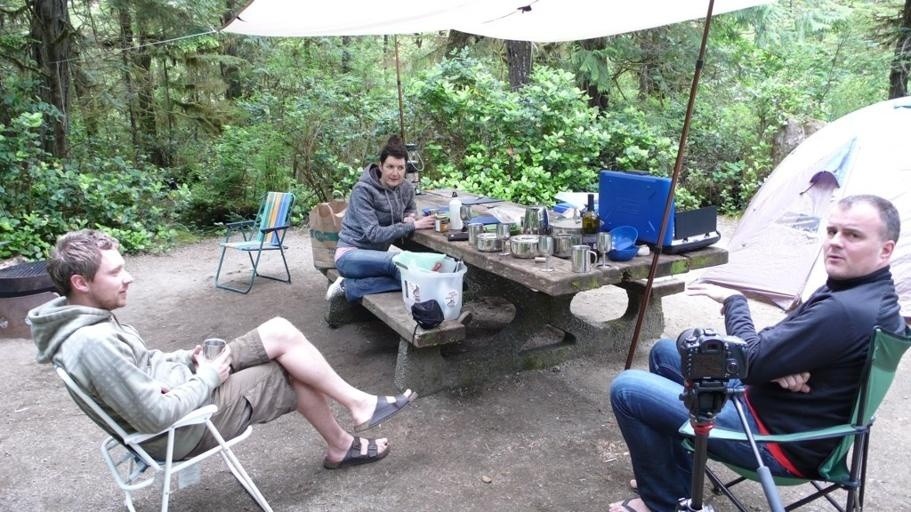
[326,135,475,327]
[607,193,909,511]
[26,229,419,471]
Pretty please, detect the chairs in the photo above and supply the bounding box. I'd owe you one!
[214,190,298,296]
[679,324,911,512]
[51,364,293,512]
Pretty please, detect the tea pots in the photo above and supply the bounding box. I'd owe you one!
[524,206,548,235]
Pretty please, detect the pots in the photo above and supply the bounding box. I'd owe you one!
[548,216,606,245]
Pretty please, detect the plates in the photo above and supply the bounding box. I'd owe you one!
[606,246,639,261]
[609,226,639,248]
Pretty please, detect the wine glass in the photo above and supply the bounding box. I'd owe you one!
[596,231,612,269]
[538,237,554,273]
[496,222,511,256]
[459,205,471,230]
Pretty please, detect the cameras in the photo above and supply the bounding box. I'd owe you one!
[676,326,750,383]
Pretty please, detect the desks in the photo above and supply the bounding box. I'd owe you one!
[397,184,730,367]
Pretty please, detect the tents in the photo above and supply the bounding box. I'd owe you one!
[706,93,909,330]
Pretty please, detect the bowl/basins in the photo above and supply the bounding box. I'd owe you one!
[508,235,540,258]
[475,232,503,252]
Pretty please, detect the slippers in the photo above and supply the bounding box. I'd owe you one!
[323,435,390,469]
[355,392,418,431]
[609,495,671,511]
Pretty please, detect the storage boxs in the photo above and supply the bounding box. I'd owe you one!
[308,199,349,270]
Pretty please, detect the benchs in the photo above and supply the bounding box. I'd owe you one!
[611,275,687,353]
[324,264,469,401]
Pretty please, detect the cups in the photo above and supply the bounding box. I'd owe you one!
[570,245,598,273]
[468,223,487,247]
[202,337,226,359]
[430,209,442,216]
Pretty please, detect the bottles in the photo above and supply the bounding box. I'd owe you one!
[449,191,463,230]
[440,221,449,233]
[581,193,599,263]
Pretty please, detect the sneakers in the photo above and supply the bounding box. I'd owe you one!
[325,276,344,302]
